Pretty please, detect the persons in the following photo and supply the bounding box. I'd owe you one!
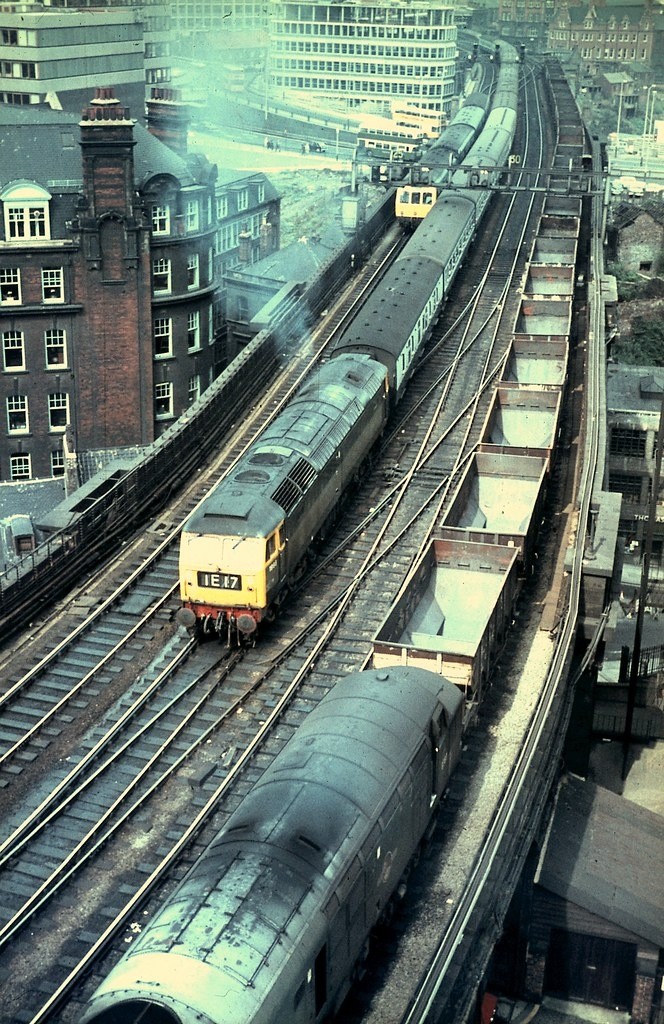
[263,127,326,156]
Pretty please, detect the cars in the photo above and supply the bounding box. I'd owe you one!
[301,141,327,153]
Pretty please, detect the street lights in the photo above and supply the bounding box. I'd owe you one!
[614,79,628,157]
[640,85,657,165]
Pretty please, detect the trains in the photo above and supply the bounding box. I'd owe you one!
[73,31,594,1024]
[176,31,521,649]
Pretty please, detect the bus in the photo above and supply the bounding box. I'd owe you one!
[356,132,424,163]
[390,103,447,139]
[220,63,244,92]
[359,120,424,139]
